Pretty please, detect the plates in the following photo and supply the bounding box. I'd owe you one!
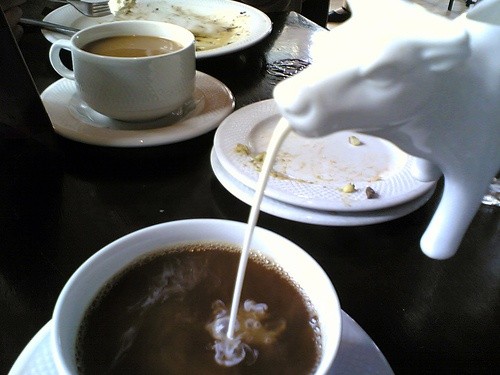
[7,307,395,375]
[210,98,441,227]
[41,0,272,58]
[40,70,235,148]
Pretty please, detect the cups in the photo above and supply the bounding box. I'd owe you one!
[48,19,196,125]
[50,218,342,375]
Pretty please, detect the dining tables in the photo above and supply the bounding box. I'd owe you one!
[0,10,500,375]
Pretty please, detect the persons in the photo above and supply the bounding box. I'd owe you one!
[0,0,27,42]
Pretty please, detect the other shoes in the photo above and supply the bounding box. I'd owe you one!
[328,8,351,22]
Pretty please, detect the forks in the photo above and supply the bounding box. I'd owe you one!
[49,0,113,17]
